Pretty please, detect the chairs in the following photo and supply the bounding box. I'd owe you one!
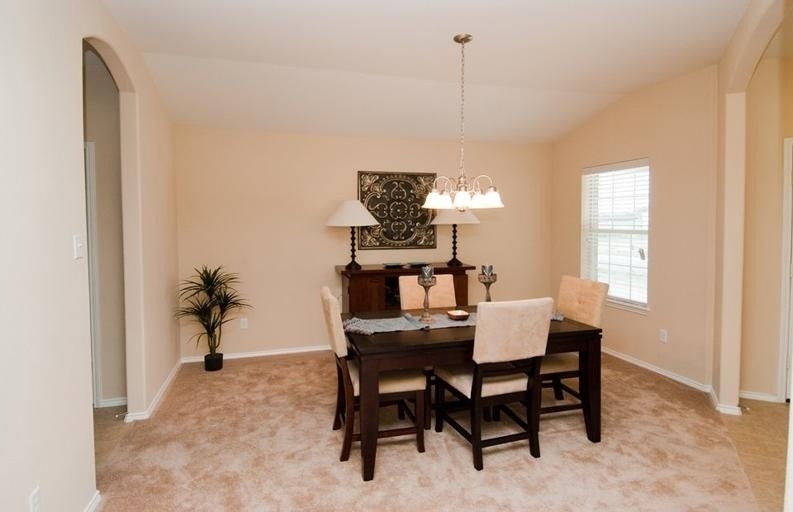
[434,296,555,472]
[319,287,428,462]
[396,273,459,431]
[519,273,613,405]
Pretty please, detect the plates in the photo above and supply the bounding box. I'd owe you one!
[383,262,407,267]
[407,262,430,267]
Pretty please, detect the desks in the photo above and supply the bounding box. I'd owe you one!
[335,304,603,483]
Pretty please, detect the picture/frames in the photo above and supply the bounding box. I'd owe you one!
[356,171,436,250]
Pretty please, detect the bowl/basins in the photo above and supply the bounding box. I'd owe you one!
[446,309,470,319]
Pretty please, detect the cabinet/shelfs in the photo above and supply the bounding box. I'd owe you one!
[332,262,476,313]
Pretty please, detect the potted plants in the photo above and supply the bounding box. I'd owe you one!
[167,264,253,371]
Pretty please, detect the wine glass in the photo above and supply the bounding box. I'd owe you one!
[418,276,438,324]
[477,274,497,302]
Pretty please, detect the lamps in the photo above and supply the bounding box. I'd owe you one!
[418,33,506,211]
[427,207,481,266]
[322,198,381,271]
